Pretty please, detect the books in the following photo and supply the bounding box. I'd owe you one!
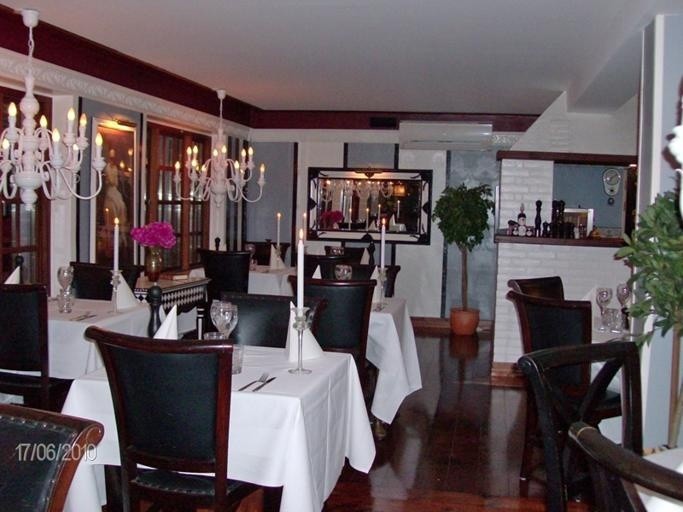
[160,274,189,281]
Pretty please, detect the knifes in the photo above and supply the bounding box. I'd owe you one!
[76,314,97,322]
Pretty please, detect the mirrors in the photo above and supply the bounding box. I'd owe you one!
[306,165,434,247]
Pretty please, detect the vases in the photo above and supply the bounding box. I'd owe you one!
[146,245,164,282]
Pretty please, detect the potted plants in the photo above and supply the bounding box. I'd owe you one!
[430,181,496,336]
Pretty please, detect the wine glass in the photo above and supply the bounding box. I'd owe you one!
[595,288,613,332]
[335,265,345,279]
[210,299,232,333]
[245,244,256,264]
[250,259,258,271]
[617,284,630,309]
[215,304,239,339]
[341,266,352,281]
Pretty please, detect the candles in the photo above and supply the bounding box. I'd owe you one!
[377,203,382,227]
[297,227,305,319]
[380,217,386,269]
[276,212,281,247]
[113,216,119,272]
[348,207,352,231]
[302,211,307,247]
[397,199,400,222]
[365,207,370,231]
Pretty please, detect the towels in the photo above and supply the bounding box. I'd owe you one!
[116,272,138,309]
[152,304,179,339]
[270,244,286,271]
[285,300,322,364]
[370,265,382,304]
[312,264,322,279]
[369,220,376,230]
[4,266,20,285]
[389,214,395,225]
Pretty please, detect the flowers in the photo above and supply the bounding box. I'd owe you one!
[129,221,178,250]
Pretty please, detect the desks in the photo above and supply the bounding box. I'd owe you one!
[133,273,212,314]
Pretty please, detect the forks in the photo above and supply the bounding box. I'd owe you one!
[69,311,92,321]
[238,372,270,391]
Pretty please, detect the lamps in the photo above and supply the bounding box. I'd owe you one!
[0,9,108,211]
[173,90,265,208]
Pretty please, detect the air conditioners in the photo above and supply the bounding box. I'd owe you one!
[398,119,494,154]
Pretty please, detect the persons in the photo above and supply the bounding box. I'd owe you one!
[104,150,126,248]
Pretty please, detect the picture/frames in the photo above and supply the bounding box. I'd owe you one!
[89,115,140,265]
[556,207,595,239]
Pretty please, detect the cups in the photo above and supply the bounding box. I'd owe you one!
[58,266,74,304]
[232,344,244,374]
[60,290,75,313]
[611,310,626,332]
[204,332,223,340]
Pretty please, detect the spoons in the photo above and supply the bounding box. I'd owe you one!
[252,377,276,392]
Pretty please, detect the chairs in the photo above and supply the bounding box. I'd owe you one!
[194,246,254,333]
[0,402,105,512]
[218,288,292,351]
[506,289,622,500]
[245,240,291,267]
[517,340,644,512]
[82,324,270,512]
[1,282,64,409]
[507,276,565,300]
[564,419,683,511]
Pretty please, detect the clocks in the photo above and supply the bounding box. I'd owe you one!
[602,167,623,206]
